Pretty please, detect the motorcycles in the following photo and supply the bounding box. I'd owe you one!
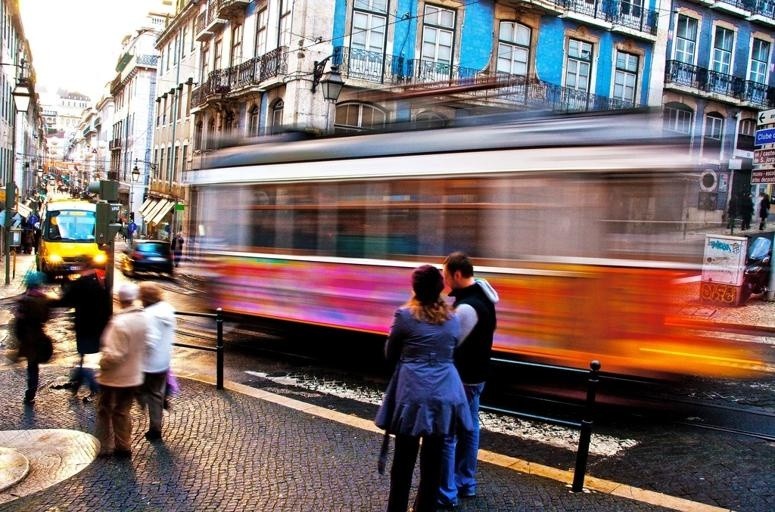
[736,232,772,306]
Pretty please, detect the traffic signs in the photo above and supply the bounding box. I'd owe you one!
[757,108,775,127]
[750,169,775,184]
[755,128,775,146]
[751,149,775,165]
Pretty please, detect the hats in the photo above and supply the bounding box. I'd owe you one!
[138,288,161,304]
[23,273,44,288]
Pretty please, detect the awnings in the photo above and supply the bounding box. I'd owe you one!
[139,197,174,227]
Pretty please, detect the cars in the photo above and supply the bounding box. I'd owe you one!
[118,238,175,278]
[47,175,54,185]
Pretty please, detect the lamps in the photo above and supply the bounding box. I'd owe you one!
[0,58,33,115]
[311,47,345,102]
[132,157,159,181]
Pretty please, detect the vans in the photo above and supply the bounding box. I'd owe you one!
[36,198,109,279]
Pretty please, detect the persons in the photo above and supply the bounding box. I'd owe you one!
[8,269,54,403]
[438,250,500,506]
[726,191,771,230]
[137,280,176,440]
[119,208,137,244]
[171,234,184,268]
[48,253,112,403]
[20,179,48,255]
[374,265,474,512]
[94,282,147,460]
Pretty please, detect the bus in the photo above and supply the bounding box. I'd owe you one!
[169,113,744,417]
